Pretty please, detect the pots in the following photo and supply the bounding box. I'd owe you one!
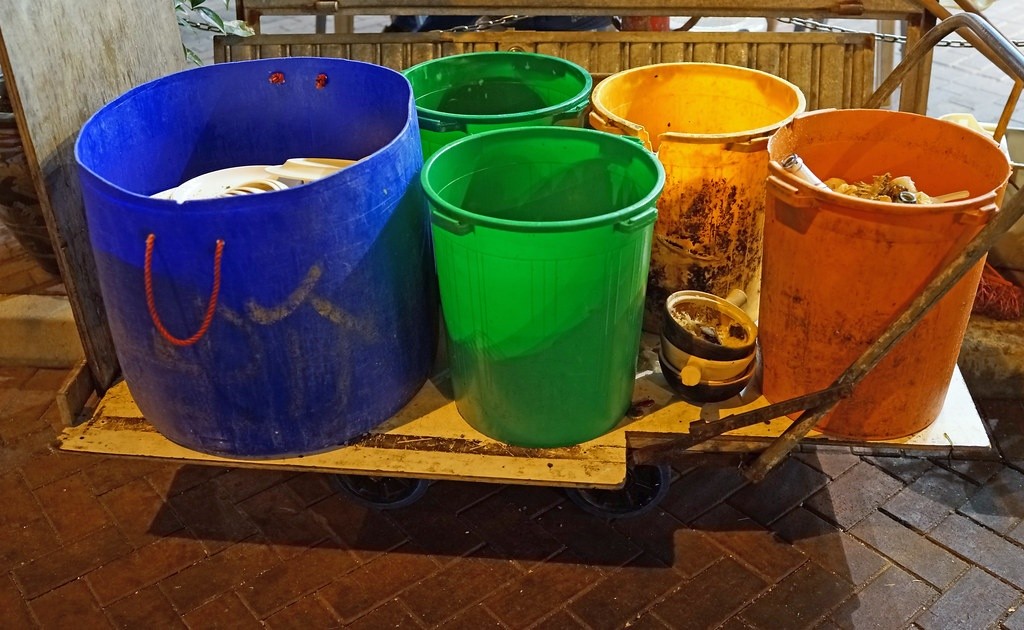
[660,289,757,405]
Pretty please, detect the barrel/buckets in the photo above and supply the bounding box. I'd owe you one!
[74,55,440,459]
[588,60,807,333]
[419,126,668,448]
[400,50,594,164]
[760,107,1013,440]
